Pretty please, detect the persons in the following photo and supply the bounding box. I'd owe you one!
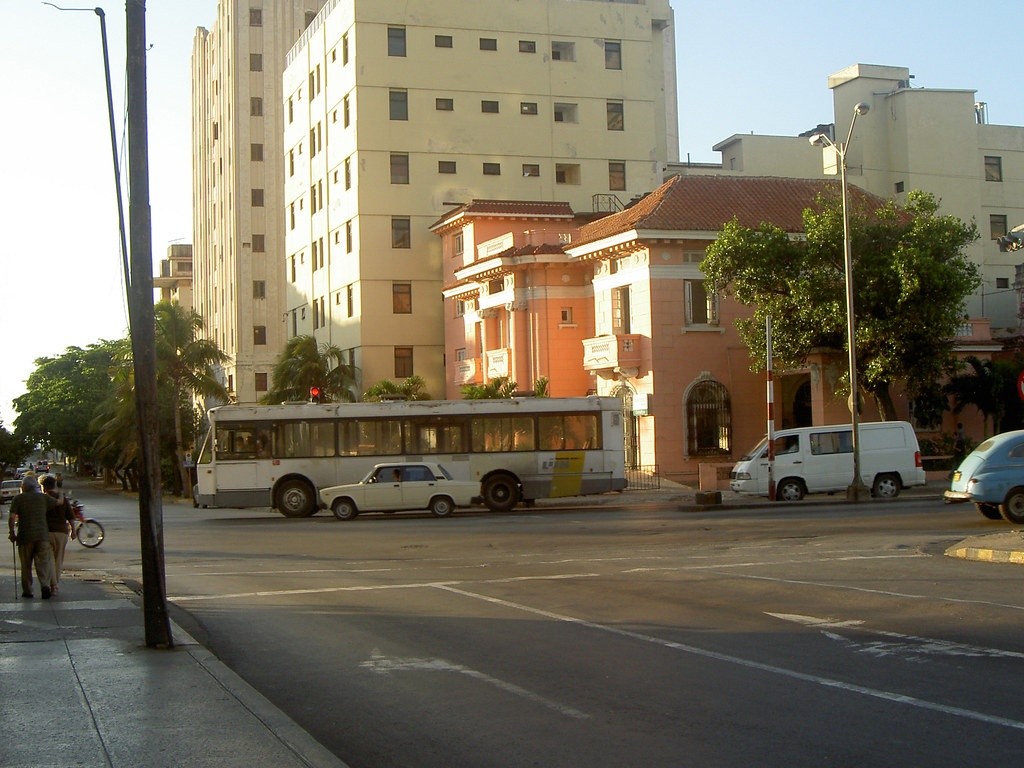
[7,471,77,599]
[224,436,250,460]
[783,437,798,454]
[391,468,400,481]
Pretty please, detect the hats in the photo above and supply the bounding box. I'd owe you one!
[22,476,37,487]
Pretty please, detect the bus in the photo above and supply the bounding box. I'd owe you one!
[190,394,630,518]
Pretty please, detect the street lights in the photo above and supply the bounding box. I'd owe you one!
[807,101,873,501]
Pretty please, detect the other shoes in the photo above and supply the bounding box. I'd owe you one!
[56,572,61,577]
[51,585,57,596]
[42,592,52,599]
[22,592,34,598]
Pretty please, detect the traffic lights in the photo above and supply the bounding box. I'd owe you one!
[309,387,320,403]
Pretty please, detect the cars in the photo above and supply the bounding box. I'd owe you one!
[317,463,484,521]
[941,431,1024,525]
[15,468,33,480]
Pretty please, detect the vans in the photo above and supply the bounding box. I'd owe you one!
[34,460,50,473]
[729,419,927,506]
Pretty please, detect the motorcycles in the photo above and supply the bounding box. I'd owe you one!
[13,489,105,549]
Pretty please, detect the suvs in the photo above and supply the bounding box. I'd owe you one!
[0,479,24,505]
